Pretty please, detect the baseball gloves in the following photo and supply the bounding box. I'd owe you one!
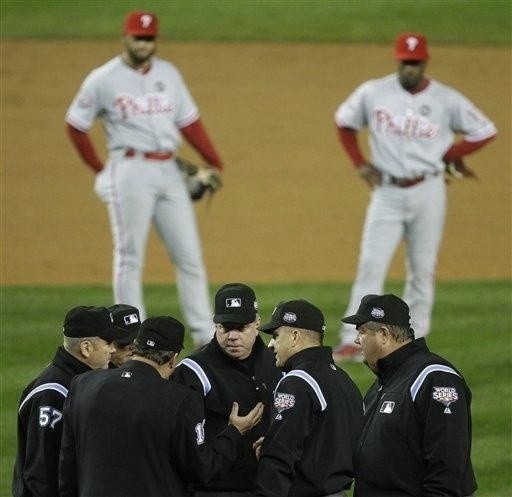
[178,162,220,200]
[445,158,473,182]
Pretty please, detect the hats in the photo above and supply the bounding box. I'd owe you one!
[134,316,185,351]
[342,295,410,326]
[260,298,327,334]
[62,303,130,339]
[213,283,256,327]
[108,304,140,345]
[396,33,429,61]
[123,12,158,37]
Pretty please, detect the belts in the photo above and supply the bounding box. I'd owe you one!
[126,146,172,160]
[391,177,424,186]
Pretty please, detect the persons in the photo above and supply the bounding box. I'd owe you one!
[251,299,365,497]
[340,293,477,497]
[58,316,265,497]
[105,304,141,369]
[169,282,283,497]
[64,10,223,353]
[12,306,130,497]
[330,33,498,366]
